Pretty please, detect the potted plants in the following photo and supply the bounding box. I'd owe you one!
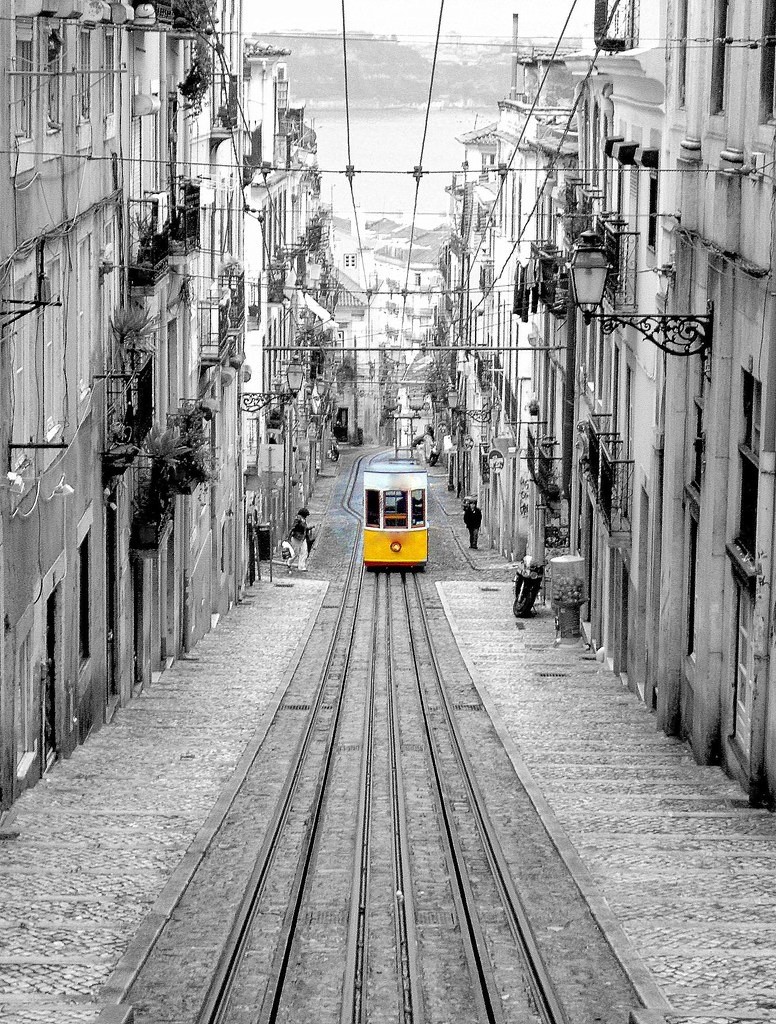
[121,208,170,283]
[122,407,211,553]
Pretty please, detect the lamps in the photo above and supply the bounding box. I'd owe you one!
[8,473,25,495]
[54,483,74,497]
[446,386,492,423]
[237,354,306,412]
[566,227,715,364]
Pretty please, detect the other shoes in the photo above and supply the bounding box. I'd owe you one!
[287,563,291,568]
[301,570,308,572]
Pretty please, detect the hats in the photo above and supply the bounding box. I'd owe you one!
[298,508,310,516]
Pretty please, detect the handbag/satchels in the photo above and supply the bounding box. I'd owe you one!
[282,541,295,559]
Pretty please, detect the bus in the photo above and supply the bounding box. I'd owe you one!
[363,459,430,572]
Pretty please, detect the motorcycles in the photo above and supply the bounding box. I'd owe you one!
[512,555,550,618]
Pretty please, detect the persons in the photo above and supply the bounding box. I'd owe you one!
[285,508,315,574]
[396,491,422,528]
[463,499,482,550]
